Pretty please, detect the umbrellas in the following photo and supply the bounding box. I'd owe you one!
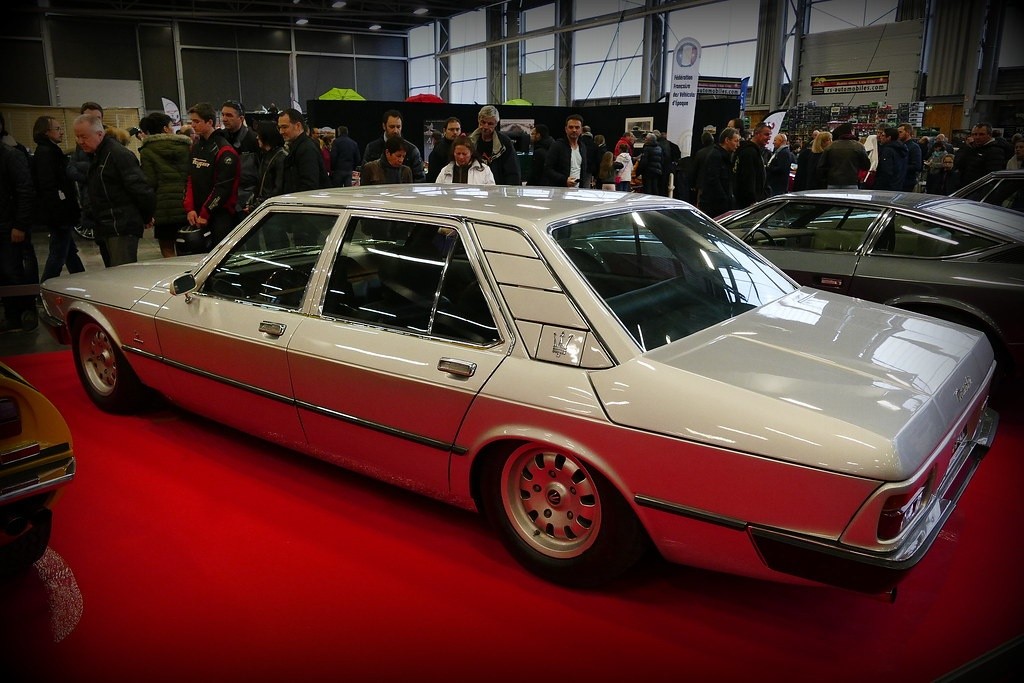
[319,88,367,101]
[406,94,444,103]
[503,99,532,105]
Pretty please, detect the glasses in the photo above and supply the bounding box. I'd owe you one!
[48,127,64,132]
[944,161,953,164]
[225,99,243,114]
[758,122,773,129]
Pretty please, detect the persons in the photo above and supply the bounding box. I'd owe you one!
[0,100,1024,334]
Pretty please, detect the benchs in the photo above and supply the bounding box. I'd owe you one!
[603,275,692,326]
[912,228,951,257]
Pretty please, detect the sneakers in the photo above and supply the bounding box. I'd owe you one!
[0,309,40,335]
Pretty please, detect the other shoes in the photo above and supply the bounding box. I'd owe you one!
[36,299,45,314]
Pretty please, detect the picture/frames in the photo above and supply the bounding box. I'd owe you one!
[625,117,653,148]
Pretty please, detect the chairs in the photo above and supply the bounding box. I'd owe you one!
[351,243,451,324]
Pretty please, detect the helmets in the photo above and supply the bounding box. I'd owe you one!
[174,225,214,256]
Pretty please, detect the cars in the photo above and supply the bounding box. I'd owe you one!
[36,181,1004,597]
[947,171,1024,216]
[710,189,1024,411]
